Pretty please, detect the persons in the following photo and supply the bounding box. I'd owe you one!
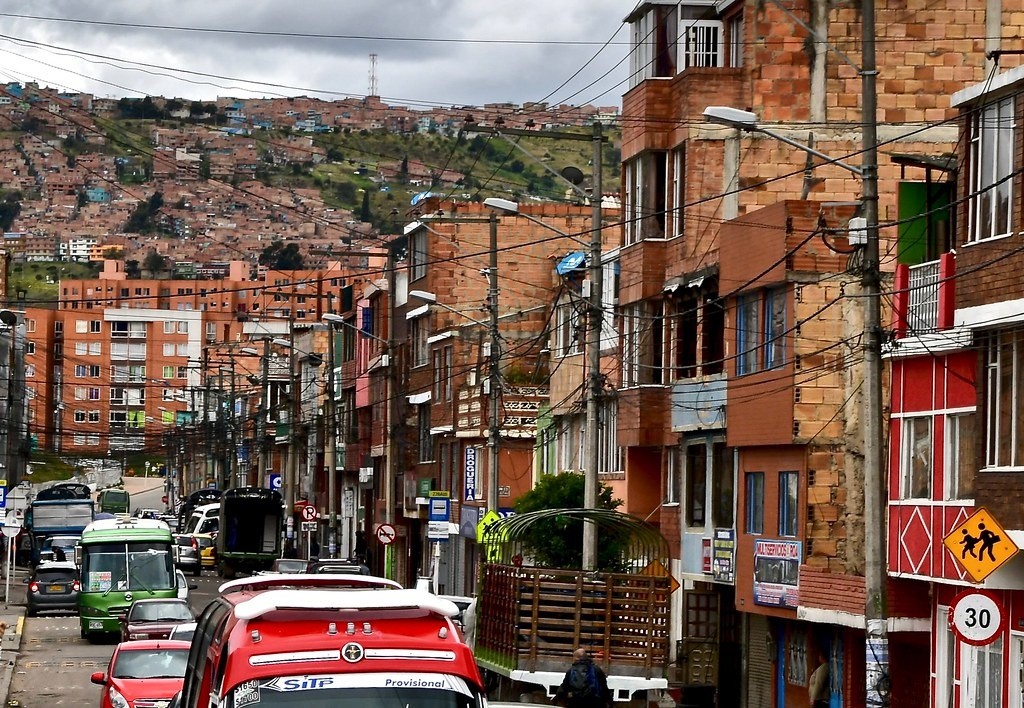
[807,650,831,708]
[310,536,320,562]
[352,530,367,560]
[549,648,614,708]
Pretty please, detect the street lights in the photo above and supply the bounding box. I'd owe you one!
[271,338,337,560]
[320,316,397,591]
[241,347,297,559]
[407,289,502,515]
[698,104,893,708]
[480,194,606,579]
[217,366,269,488]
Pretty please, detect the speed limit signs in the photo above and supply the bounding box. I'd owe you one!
[948,586,1007,648]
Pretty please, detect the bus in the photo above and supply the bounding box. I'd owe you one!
[179,573,486,708]
[70,513,181,639]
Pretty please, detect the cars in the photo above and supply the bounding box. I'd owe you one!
[23,482,372,590]
[117,598,197,641]
[175,569,199,598]
[89,638,193,708]
[150,620,198,668]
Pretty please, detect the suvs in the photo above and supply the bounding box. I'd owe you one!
[23,560,79,617]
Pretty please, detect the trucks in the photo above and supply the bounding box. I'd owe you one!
[459,506,673,708]
